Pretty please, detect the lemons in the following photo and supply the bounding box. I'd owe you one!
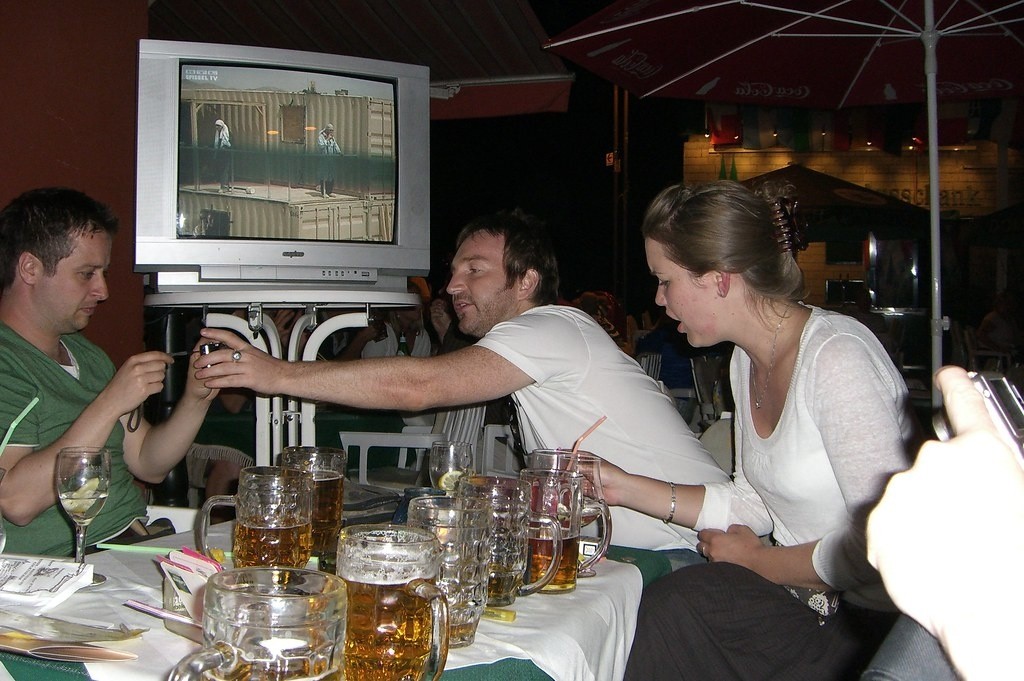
[66,478,99,510]
[438,471,466,492]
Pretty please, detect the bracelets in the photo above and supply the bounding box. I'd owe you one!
[663,482,676,523]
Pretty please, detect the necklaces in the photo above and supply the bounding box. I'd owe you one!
[751,305,789,409]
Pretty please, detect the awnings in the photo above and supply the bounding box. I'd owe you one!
[147,0,576,118]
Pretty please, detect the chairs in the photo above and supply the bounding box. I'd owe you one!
[139,312,1024,532]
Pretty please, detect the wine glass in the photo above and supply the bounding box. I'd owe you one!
[55,445,111,587]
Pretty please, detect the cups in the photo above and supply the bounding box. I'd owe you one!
[429,440,473,495]
[520,447,612,595]
[194,467,313,570]
[282,446,345,552]
[336,523,449,680]
[457,475,564,604]
[167,567,348,681]
[409,496,491,648]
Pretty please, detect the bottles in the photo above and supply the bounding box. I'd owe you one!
[392,486,446,528]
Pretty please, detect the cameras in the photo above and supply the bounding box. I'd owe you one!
[200,343,229,369]
[930,369,1024,472]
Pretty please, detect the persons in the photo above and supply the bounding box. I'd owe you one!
[194,215,731,571]
[976,291,1024,369]
[556,181,920,681]
[212,119,233,194]
[0,188,224,557]
[220,284,628,474]
[318,123,342,198]
[842,284,899,357]
[866,365,1024,681]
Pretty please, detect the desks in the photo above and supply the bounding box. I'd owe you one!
[144,293,421,467]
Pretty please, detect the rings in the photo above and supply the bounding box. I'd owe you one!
[700,545,707,556]
[232,349,242,362]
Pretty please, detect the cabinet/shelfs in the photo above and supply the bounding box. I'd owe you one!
[0,510,673,681]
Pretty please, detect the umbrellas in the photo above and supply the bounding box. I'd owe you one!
[542,0,1024,413]
[742,164,921,224]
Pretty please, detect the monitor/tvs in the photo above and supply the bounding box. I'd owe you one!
[136,39,431,304]
